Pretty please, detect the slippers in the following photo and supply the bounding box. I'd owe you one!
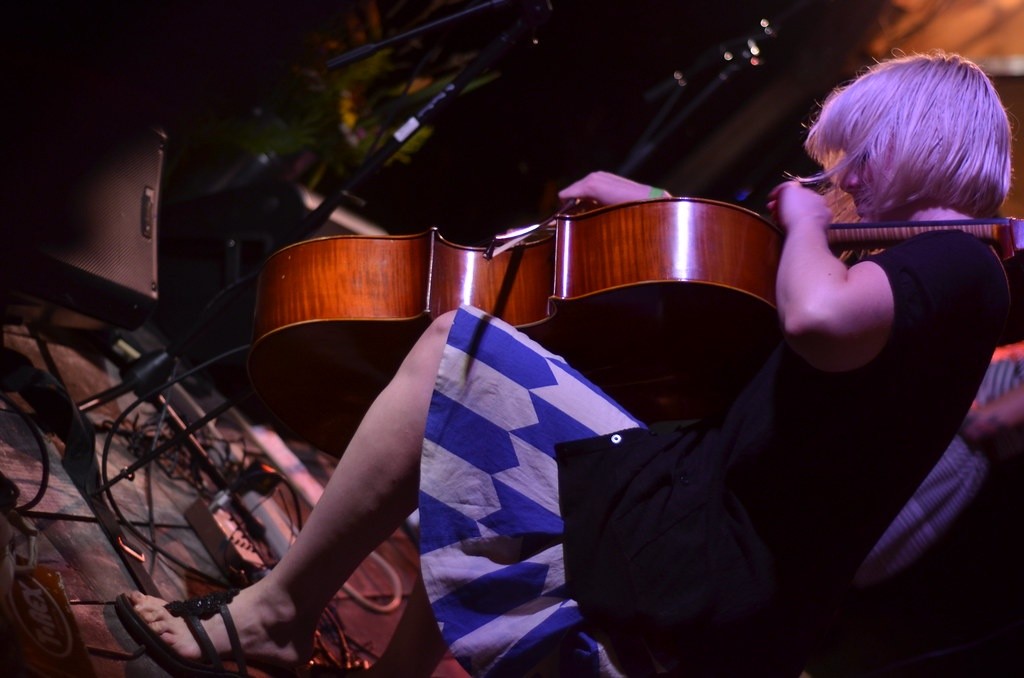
[115,590,296,678]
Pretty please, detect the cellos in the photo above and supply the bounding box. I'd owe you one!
[94,193,1020,481]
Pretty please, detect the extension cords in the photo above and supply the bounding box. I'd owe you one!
[185,497,264,591]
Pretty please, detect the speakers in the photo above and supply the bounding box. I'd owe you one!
[0,109,392,381]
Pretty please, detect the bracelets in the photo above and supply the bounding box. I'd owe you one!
[650,186,664,198]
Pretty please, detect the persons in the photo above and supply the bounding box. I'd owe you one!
[846,338,1024,590]
[114,52,1014,677]
[162,36,478,197]
